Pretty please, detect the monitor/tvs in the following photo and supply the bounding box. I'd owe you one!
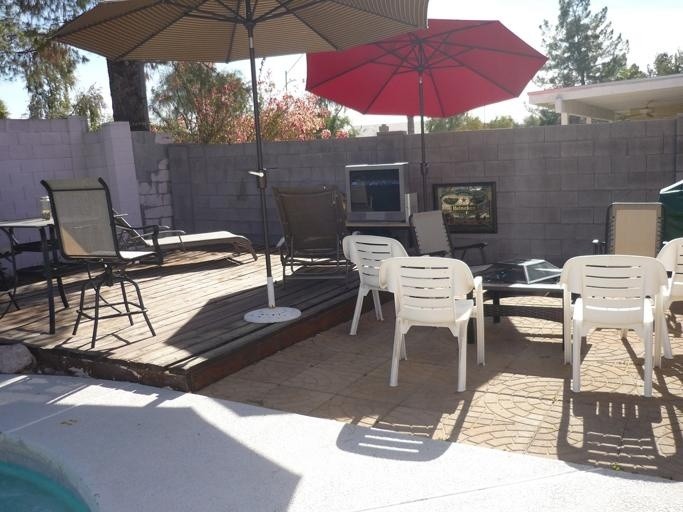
[345,161,408,221]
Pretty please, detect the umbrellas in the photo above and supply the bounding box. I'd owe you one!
[303,19,548,211]
[46,0,432,307]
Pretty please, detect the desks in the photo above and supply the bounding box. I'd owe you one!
[464,260,583,347]
[1,212,130,334]
[341,218,412,255]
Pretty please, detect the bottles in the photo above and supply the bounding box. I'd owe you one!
[39,195,50,220]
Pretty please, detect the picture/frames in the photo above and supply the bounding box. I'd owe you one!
[430,180,498,235]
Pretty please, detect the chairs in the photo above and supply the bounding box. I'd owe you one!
[271,183,354,292]
[339,233,410,336]
[651,236,683,363]
[407,209,487,275]
[556,253,668,399]
[39,176,162,347]
[105,199,256,266]
[379,253,485,393]
[588,200,670,258]
